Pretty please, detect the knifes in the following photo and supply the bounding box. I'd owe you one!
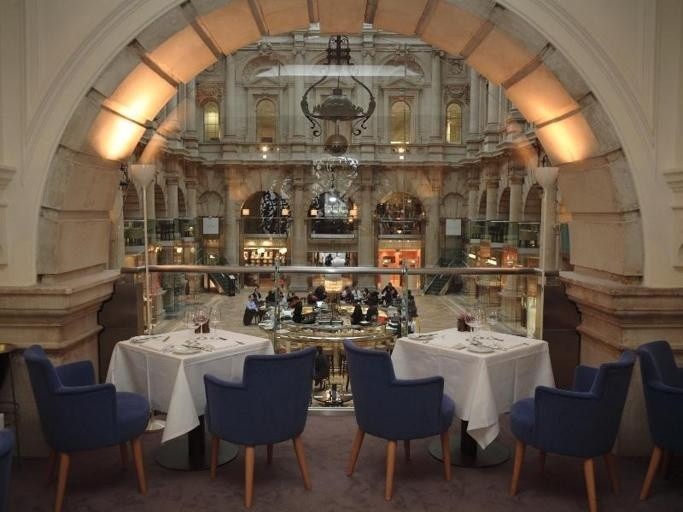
[219,337,245,345]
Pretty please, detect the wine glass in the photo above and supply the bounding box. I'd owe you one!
[464,309,498,342]
[185,310,221,341]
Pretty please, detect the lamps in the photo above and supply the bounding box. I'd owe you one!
[535,167,558,342]
[131,164,155,328]
[301,35,376,137]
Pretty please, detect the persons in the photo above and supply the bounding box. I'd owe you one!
[241,281,419,337]
[323,253,333,267]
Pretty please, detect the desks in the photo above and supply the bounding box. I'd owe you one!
[125,228,143,246]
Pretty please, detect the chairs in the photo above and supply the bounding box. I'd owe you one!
[160,220,174,241]
[204,347,317,510]
[0,426,17,512]
[636,340,683,500]
[23,344,149,512]
[510,351,637,512]
[343,339,454,502]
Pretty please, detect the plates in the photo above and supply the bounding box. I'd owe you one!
[408,332,433,340]
[466,344,495,353]
[173,346,201,354]
[129,335,156,344]
